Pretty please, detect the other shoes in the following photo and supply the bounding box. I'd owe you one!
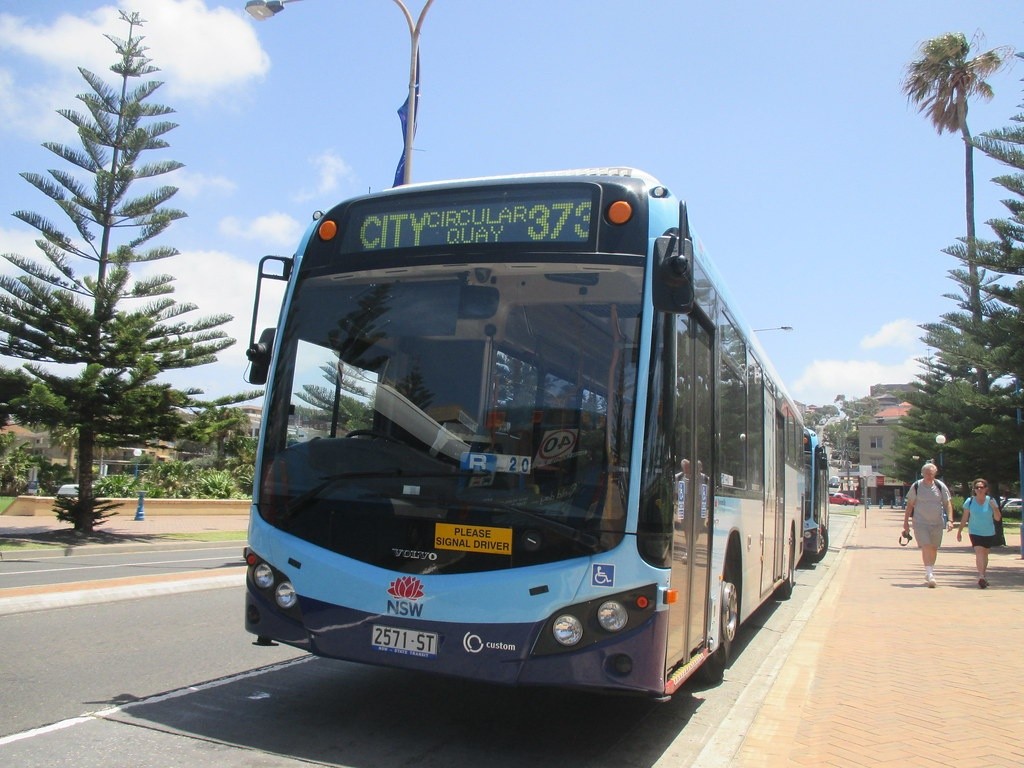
[978,577,990,588]
[925,575,936,588]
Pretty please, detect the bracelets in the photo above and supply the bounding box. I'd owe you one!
[948,520,953,523]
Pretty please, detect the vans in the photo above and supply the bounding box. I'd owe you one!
[57,483,81,504]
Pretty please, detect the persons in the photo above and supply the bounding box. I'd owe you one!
[957,478,1002,587]
[904,463,954,587]
[676,459,709,563]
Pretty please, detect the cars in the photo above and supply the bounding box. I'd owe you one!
[1001,498,1022,515]
[830,493,860,506]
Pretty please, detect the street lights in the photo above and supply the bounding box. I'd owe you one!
[244,0,435,185]
[133,449,144,480]
[935,435,946,481]
[912,455,920,479]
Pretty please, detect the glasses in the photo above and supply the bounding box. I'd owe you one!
[974,486,985,489]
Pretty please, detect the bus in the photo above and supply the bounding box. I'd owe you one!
[243,165,807,702]
[802,427,830,561]
